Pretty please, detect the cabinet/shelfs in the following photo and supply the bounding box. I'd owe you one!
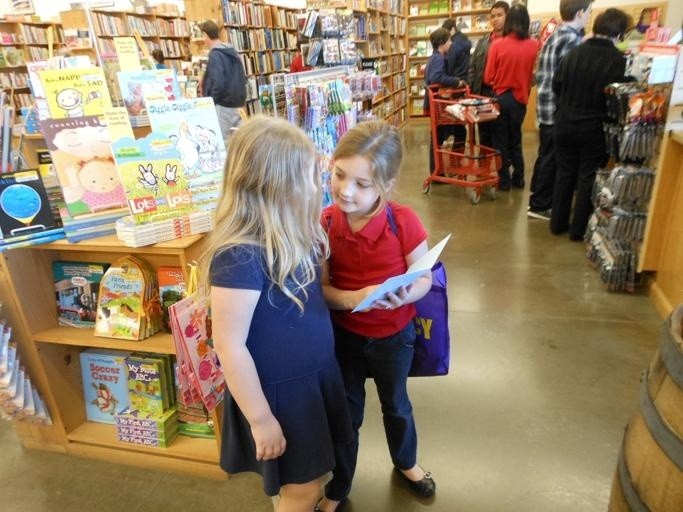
[0,226,232,482]
[636,107,682,320]
[0,0,515,175]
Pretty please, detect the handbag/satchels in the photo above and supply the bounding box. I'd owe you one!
[401,262,450,376]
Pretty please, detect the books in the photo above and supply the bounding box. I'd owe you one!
[408,2,471,56]
[354,234,451,313]
[407,59,429,113]
[0,0,410,449]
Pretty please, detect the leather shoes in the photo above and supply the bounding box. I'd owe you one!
[315,495,347,512]
[396,463,436,495]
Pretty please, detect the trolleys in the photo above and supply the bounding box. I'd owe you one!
[421,77,505,206]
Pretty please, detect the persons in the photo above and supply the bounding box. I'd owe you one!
[427,21,472,190]
[197,115,358,512]
[471,0,641,245]
[316,118,435,512]
